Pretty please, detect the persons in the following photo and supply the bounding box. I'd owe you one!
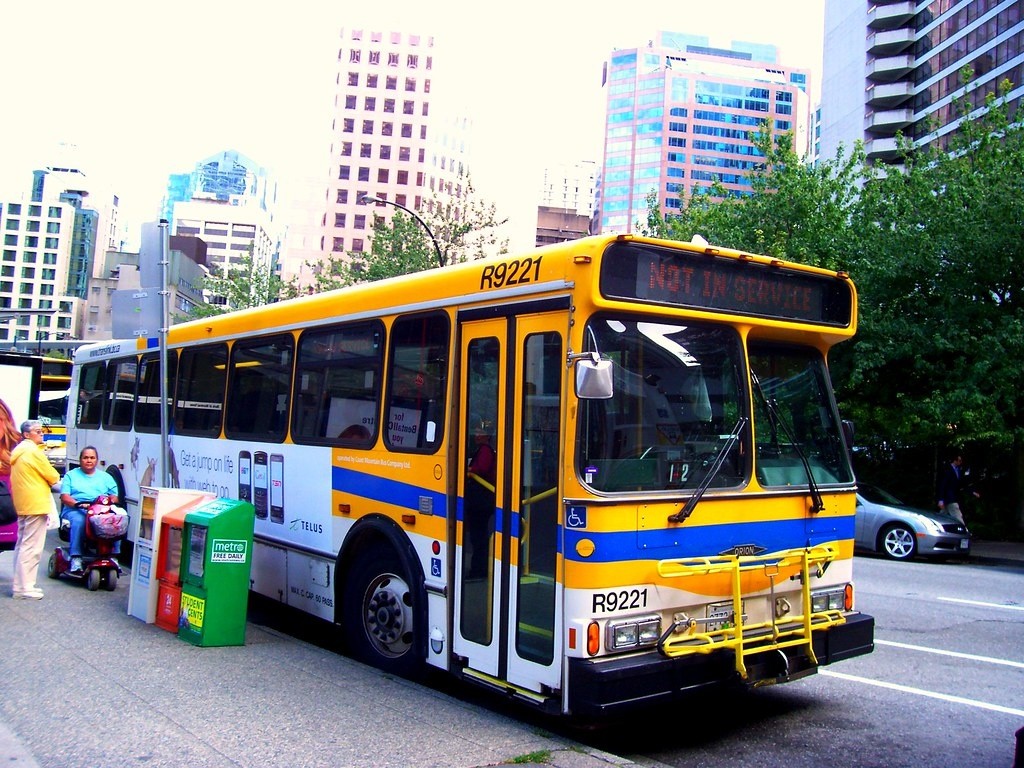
[9,419,61,599]
[936,454,980,533]
[293,367,423,438]
[464,421,496,582]
[643,331,713,442]
[59,445,124,574]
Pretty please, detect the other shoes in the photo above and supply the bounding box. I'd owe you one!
[12,588,44,600]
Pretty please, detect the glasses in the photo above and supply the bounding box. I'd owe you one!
[28,430,44,434]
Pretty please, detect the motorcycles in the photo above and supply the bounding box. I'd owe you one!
[47,495,130,591]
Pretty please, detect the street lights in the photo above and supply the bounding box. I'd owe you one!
[354,189,443,266]
[36,332,66,357]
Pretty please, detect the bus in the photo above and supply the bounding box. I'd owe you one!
[65,231,879,727]
[26,356,74,476]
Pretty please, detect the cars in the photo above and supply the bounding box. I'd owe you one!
[851,480,973,563]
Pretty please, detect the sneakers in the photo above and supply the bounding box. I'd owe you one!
[110,557,121,569]
[70,557,83,574]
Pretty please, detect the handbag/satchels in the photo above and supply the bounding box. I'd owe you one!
[46,493,61,530]
